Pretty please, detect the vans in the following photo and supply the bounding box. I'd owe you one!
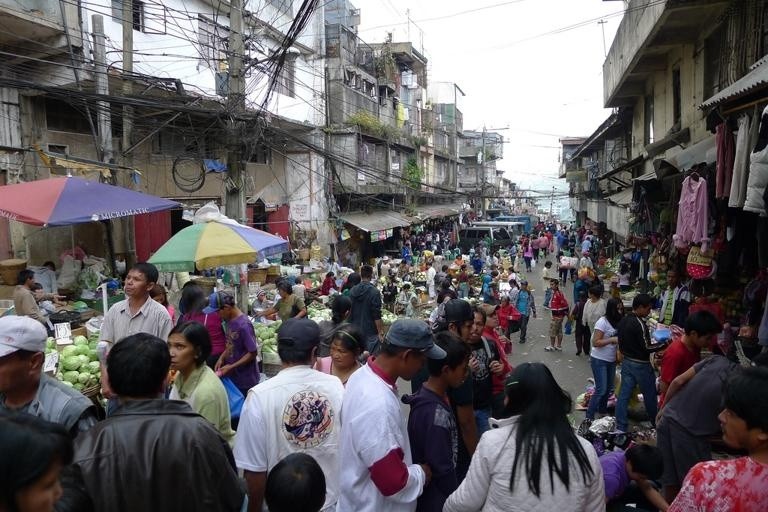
[458,226,511,254]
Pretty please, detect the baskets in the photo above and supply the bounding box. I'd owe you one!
[191,277,216,297]
[248,269,268,286]
[50,310,81,330]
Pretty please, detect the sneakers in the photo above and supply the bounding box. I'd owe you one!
[544,346,562,351]
[576,349,589,355]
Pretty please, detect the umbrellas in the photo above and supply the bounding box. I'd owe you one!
[0,171,188,267]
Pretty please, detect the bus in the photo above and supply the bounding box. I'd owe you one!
[471,216,531,243]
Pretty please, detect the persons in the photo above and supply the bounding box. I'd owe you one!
[2,215,768,510]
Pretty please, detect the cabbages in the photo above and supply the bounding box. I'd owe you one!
[252,322,278,345]
[44,330,102,389]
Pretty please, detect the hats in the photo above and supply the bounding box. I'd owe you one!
[521,280,528,285]
[278,318,320,350]
[479,303,500,315]
[256,289,266,297]
[432,299,473,332]
[0,315,47,358]
[202,291,234,314]
[384,318,447,360]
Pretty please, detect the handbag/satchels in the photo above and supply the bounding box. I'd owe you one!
[220,375,245,419]
[616,349,624,363]
[508,320,519,333]
[530,258,536,268]
[550,245,554,252]
[565,319,576,334]
[672,300,691,328]
[539,250,543,257]
[559,256,579,269]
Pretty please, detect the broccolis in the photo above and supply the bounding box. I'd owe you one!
[307,308,332,324]
[458,296,484,305]
[380,309,399,323]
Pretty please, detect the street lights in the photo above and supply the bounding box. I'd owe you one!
[236,47,301,82]
